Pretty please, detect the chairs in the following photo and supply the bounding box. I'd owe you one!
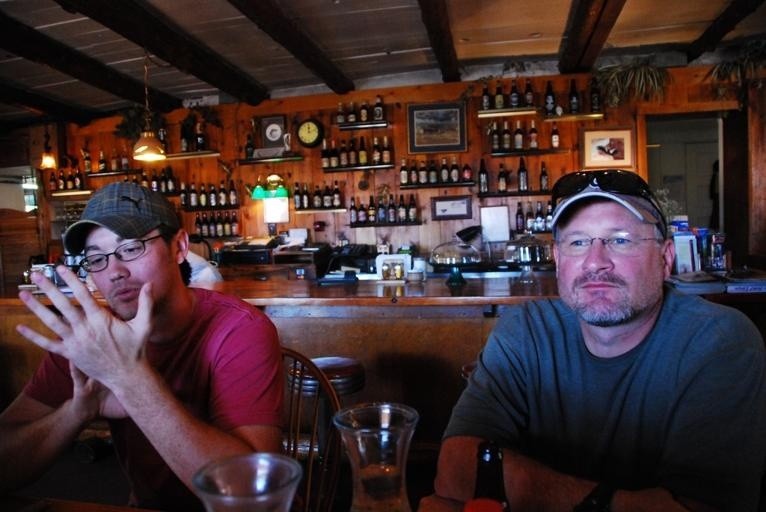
[281,347,343,511]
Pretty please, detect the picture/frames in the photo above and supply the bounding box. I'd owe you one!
[580,129,634,169]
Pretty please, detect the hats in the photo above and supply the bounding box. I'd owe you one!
[548,177,665,231]
[63,181,180,257]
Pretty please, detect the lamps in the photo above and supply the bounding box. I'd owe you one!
[133,57,164,160]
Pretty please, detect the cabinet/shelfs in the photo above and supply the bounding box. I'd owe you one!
[50,107,605,242]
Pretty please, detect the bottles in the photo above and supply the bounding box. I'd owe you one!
[516,158,530,193]
[289,177,346,210]
[535,160,551,192]
[512,199,555,233]
[19,221,92,288]
[378,214,731,286]
[49,168,84,191]
[126,165,177,194]
[192,210,239,235]
[179,123,190,153]
[329,92,390,127]
[156,125,167,156]
[473,436,511,512]
[541,79,556,119]
[391,154,477,186]
[563,77,582,115]
[193,120,205,152]
[476,158,492,193]
[478,76,539,110]
[341,187,418,226]
[588,78,603,116]
[496,162,511,192]
[180,180,240,206]
[330,402,417,512]
[316,134,396,169]
[96,146,129,174]
[486,119,543,150]
[83,147,93,175]
[550,120,563,152]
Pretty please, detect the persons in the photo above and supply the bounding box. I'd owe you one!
[0,182,285,512]
[433,168,766,512]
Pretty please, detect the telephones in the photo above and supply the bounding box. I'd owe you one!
[330,243,368,258]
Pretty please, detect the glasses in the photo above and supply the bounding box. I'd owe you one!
[80,234,160,272]
[557,233,661,256]
[550,169,667,233]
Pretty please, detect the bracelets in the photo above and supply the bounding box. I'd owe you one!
[575,480,619,512]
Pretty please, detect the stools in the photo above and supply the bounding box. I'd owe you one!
[289,357,364,474]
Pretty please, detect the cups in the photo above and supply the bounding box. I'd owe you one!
[395,287,403,297]
[383,286,391,298]
[189,452,303,512]
